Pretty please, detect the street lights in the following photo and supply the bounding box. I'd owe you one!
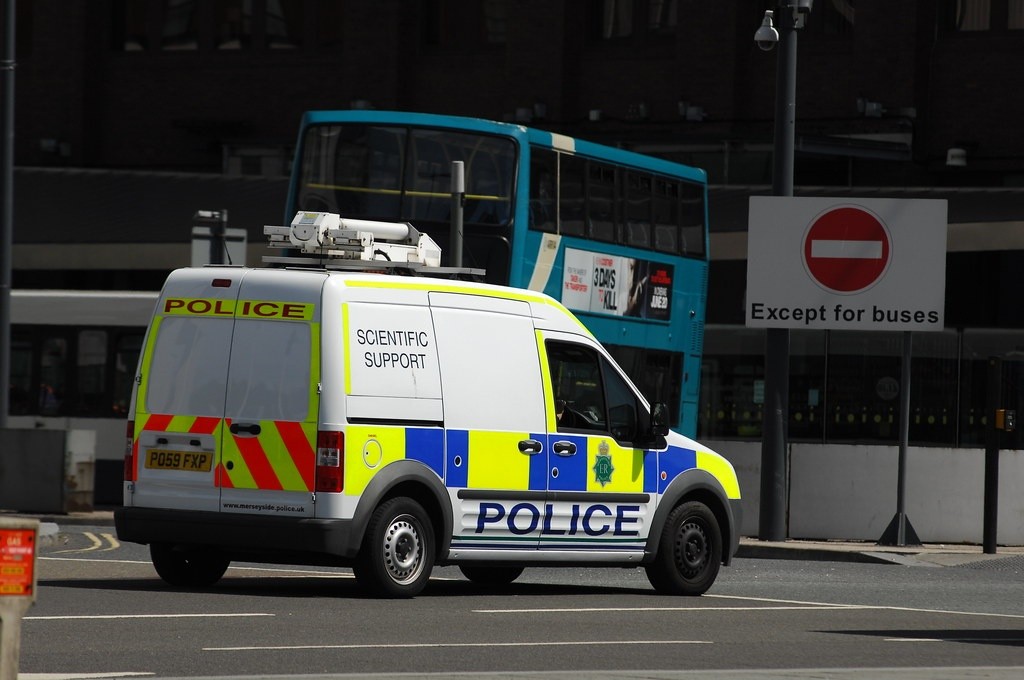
[753,0,812,544]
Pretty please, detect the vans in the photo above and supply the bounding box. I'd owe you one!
[112,212,742,598]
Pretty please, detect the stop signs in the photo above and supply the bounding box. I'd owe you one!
[803,207,890,294]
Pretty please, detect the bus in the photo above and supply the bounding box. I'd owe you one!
[282,110,713,444]
